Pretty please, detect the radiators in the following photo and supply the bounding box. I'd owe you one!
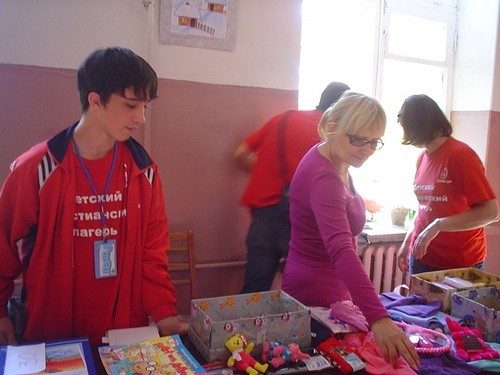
[359,244,411,297]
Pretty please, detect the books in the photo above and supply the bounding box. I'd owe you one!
[98,334,208,375]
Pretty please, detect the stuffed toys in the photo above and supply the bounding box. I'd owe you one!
[262,341,311,366]
[223,333,268,375]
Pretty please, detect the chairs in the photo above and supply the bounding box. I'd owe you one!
[167,231,199,314]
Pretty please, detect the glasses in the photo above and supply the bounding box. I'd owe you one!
[345,133,385,151]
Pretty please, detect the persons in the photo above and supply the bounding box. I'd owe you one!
[281,91,422,372]
[1,45,192,349]
[396,95,500,276]
[231,82,352,294]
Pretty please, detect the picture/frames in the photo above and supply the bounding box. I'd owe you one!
[159,0,237,51]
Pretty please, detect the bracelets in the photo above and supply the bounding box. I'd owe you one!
[435,217,440,232]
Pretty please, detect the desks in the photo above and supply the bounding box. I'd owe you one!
[92,293,500,375]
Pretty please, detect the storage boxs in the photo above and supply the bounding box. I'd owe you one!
[186,290,311,364]
[451,287,500,341]
[410,267,500,311]
